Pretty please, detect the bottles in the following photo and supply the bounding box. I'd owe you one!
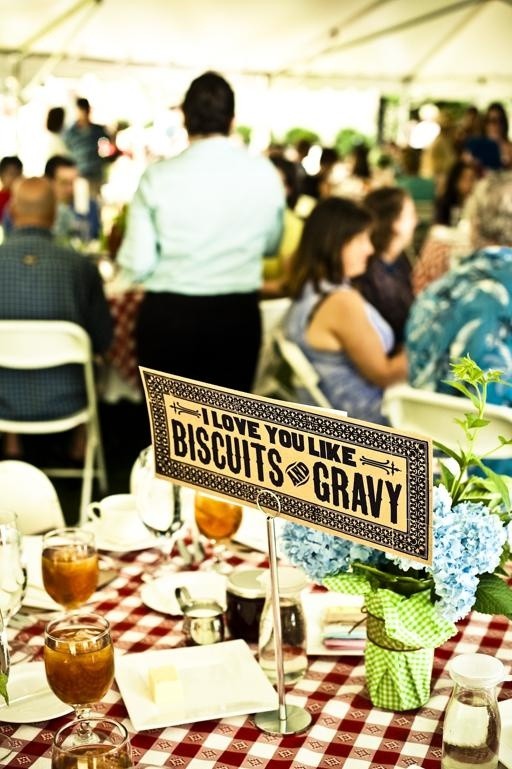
[440,653,507,769]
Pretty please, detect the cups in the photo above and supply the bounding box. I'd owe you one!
[171,586,227,647]
[40,524,102,610]
[86,494,145,538]
[225,566,327,688]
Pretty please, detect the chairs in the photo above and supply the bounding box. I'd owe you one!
[272,329,333,408]
[0,318,111,525]
[381,382,510,446]
[0,458,67,536]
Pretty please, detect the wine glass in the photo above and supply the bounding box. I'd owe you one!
[196,492,248,559]
[1,507,25,675]
[45,611,132,769]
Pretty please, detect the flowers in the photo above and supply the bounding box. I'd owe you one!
[285,351,511,628]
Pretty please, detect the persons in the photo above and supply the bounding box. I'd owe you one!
[111,67,293,400]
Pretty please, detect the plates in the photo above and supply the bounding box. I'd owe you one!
[77,521,179,551]
[138,570,235,620]
[1,659,77,724]
[111,640,280,731]
[493,696,512,769]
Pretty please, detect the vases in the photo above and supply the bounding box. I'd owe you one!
[346,558,442,708]
